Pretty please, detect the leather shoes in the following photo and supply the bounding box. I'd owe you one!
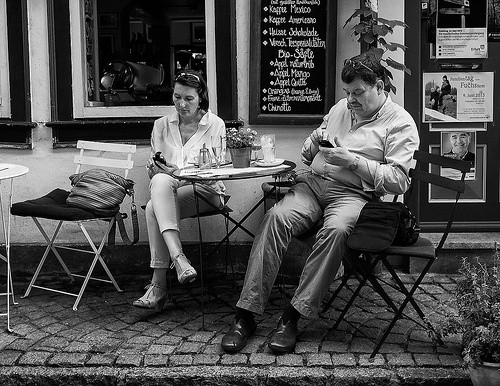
[221,313,257,352]
[268,315,298,351]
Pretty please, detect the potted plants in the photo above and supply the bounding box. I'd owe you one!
[262,170,317,276]
[424,240,500,386]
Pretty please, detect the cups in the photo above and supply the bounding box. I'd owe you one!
[261,134,276,163]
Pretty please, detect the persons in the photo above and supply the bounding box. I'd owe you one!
[439,76,451,106]
[221,53,419,353]
[429,88,439,111]
[132,72,227,308]
[442,132,475,173]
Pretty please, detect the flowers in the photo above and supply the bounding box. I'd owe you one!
[224,124,262,151]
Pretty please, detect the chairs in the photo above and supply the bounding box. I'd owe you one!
[10,140,137,313]
[322,150,473,358]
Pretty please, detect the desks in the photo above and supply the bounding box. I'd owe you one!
[173,159,298,331]
[0,163,29,332]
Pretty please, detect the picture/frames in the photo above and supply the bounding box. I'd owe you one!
[98,11,119,29]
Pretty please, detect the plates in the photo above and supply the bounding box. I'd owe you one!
[254,158,285,167]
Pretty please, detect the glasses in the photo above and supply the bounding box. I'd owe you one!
[174,72,201,85]
[343,58,374,73]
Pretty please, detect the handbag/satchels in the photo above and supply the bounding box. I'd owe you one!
[345,201,421,252]
[65,168,139,246]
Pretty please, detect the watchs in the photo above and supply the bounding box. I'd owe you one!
[350,156,360,172]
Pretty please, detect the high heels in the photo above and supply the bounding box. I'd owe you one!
[132,280,168,312]
[171,253,197,283]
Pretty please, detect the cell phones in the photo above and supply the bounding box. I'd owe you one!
[152,156,168,168]
[316,139,334,148]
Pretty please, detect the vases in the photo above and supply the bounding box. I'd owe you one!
[229,146,251,168]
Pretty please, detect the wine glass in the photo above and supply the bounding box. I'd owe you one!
[251,136,261,163]
[211,135,225,169]
